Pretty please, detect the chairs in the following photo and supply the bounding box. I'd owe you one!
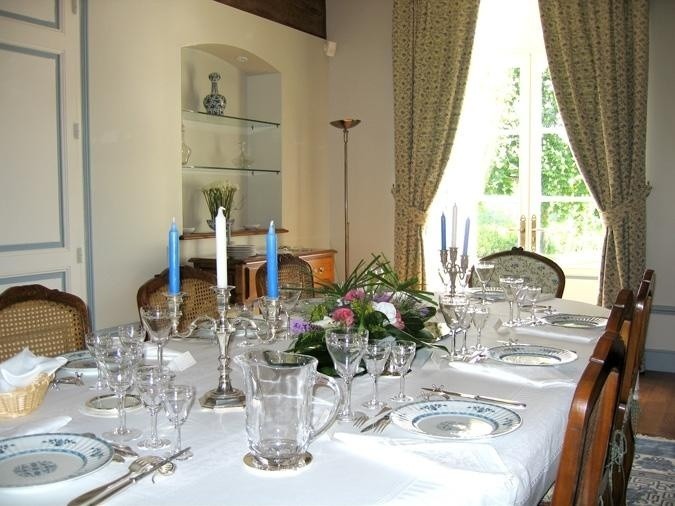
[608,288,637,506]
[539,330,629,506]
[466,247,565,298]
[0,282,90,362]
[630,268,656,400]
[136,265,225,335]
[256,252,316,300]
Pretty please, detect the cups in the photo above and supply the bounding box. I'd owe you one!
[369,265,384,289]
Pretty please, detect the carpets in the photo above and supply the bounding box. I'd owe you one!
[624,431,675,506]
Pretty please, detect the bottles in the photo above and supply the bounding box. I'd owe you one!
[181,126,191,165]
[232,140,255,168]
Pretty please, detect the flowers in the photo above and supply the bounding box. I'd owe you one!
[280,250,438,356]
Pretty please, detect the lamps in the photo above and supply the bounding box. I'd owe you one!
[329,117,363,280]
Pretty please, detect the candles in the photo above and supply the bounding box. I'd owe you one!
[451,202,457,247]
[265,219,278,299]
[463,217,470,255]
[168,217,180,293]
[440,209,447,249]
[215,206,229,289]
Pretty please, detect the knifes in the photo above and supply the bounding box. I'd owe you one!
[88,446,193,506]
[420,386,526,409]
[361,405,408,432]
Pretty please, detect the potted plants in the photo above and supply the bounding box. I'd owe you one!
[202,182,238,231]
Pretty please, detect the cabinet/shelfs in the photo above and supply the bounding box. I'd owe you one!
[179,100,289,242]
[188,247,338,308]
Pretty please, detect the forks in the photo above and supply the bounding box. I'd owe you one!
[66,455,167,506]
[350,403,396,428]
[374,402,414,434]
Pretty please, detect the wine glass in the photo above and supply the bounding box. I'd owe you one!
[78,302,200,462]
[439,260,552,363]
[323,321,413,424]
[233,279,303,347]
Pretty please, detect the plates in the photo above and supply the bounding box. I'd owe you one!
[540,315,609,329]
[0,431,113,486]
[243,224,262,230]
[183,227,194,233]
[56,351,101,373]
[227,244,258,259]
[486,343,578,366]
[393,404,519,438]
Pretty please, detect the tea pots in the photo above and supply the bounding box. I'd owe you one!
[233,346,343,467]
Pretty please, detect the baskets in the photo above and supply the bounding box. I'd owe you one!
[0,374,54,418]
